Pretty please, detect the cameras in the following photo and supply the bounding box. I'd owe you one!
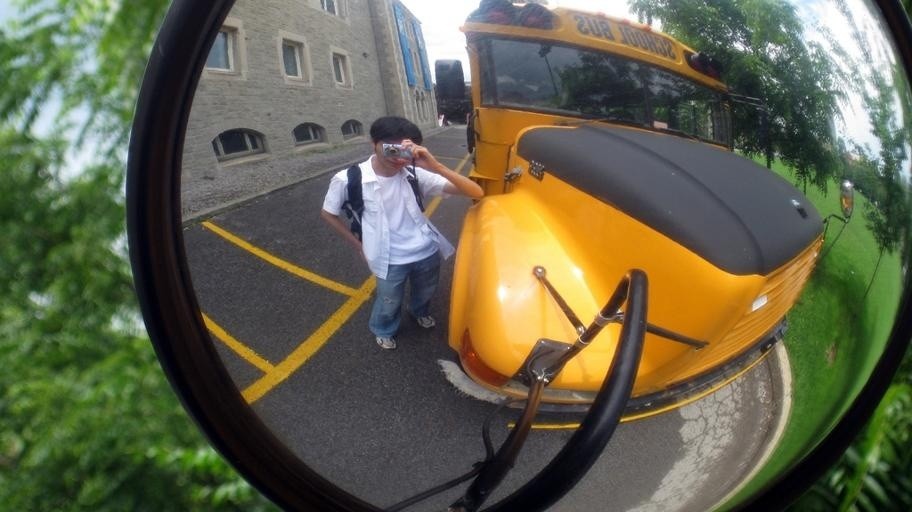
[383,143,412,157]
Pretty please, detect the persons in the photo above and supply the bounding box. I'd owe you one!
[315,114,484,351]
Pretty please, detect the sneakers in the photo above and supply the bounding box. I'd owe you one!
[377,338,396,350]
[418,315,435,329]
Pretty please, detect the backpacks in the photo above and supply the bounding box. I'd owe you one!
[343,160,426,242]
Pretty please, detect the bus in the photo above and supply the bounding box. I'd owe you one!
[435,0,855,511]
